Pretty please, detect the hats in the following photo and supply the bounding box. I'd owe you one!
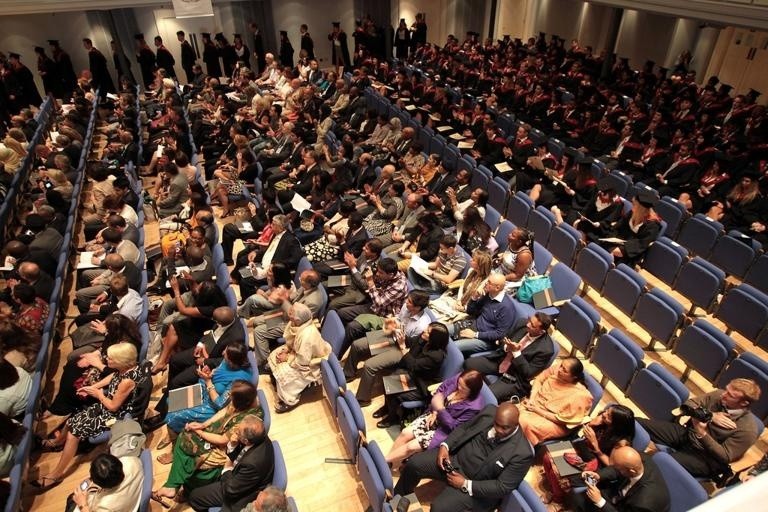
[9,12,768,209]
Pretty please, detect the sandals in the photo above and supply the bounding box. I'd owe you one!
[157,451,173,464]
[27,471,65,489]
[35,438,63,453]
[156,436,172,450]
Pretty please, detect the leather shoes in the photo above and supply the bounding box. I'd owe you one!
[372,406,388,418]
[377,414,401,429]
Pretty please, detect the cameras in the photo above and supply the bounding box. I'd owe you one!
[444,459,457,473]
[80,480,89,492]
[693,405,713,423]
[175,273,182,280]
[38,180,52,189]
[587,473,595,487]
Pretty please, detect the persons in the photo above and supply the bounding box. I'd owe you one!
[1,9,767,512]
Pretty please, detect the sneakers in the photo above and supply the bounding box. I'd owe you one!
[274,398,292,412]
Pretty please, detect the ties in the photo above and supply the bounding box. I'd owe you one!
[498,333,533,373]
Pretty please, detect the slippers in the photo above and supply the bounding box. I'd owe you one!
[150,489,171,509]
